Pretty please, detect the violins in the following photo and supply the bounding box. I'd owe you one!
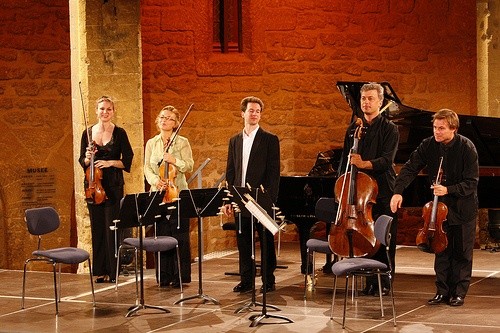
[157,137,181,204]
[415,157,448,253]
[84,142,106,204]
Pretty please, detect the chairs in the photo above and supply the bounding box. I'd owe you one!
[116,236,183,300]
[21,207,96,314]
[304,198,397,329]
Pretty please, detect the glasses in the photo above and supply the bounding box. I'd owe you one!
[159,115,176,121]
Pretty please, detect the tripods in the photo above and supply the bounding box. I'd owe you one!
[175,188,227,304]
[116,190,171,318]
[234,218,282,315]
[227,185,293,326]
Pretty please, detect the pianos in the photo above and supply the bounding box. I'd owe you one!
[276,82,500,274]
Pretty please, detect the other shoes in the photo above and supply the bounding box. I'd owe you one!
[94,277,107,282]
[110,277,116,282]
[172,282,180,287]
[161,282,170,288]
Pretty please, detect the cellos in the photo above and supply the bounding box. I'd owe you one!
[329,117,381,302]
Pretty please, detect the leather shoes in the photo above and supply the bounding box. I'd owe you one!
[375,287,390,296]
[233,282,248,291]
[260,284,275,291]
[427,294,450,304]
[450,295,465,306]
[358,285,377,295]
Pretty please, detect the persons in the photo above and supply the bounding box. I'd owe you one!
[223,96,281,294]
[389,108,479,307]
[337,82,401,298]
[142,104,196,288]
[77,95,135,284]
